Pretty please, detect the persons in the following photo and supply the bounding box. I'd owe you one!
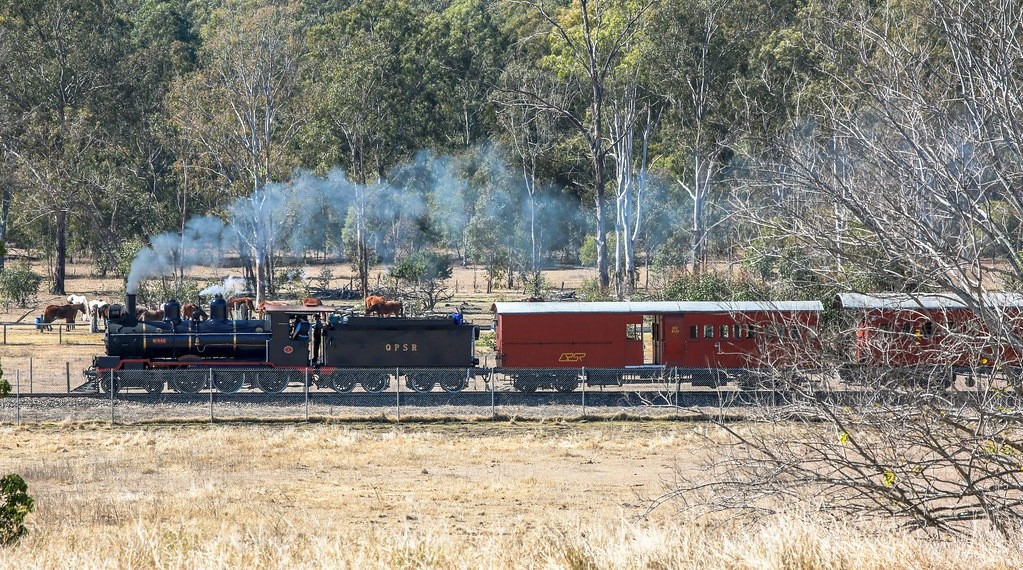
[311,313,324,364]
[291,314,312,342]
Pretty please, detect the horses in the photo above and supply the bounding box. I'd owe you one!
[365,295,403,318]
[40,294,323,332]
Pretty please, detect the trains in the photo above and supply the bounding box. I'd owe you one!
[84,286,1023,385]
